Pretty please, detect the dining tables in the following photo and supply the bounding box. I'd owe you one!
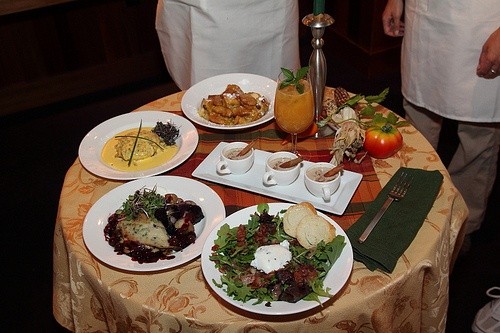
[52,86,469,333]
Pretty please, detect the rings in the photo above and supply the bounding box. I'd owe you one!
[490,68,496,74]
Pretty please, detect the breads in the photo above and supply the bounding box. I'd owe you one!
[282,202,317,239]
[296,215,336,249]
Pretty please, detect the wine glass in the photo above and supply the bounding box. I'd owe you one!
[274,69,315,157]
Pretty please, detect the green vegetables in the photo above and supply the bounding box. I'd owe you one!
[279,67,411,128]
[209,202,347,307]
[116,184,167,222]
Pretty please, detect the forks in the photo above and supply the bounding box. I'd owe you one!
[359,172,415,243]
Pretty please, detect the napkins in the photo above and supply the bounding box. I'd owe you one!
[345,167,444,274]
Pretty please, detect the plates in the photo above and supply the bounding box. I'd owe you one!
[80,110,198,181]
[82,175,225,271]
[181,73,278,129]
[191,141,364,215]
[201,202,353,314]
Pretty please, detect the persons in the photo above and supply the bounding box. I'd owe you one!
[382,0,500,235]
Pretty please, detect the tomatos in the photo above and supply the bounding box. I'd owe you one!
[365,123,403,158]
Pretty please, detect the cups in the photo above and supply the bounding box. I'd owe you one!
[215,141,254,175]
[303,162,340,201]
[263,152,300,186]
